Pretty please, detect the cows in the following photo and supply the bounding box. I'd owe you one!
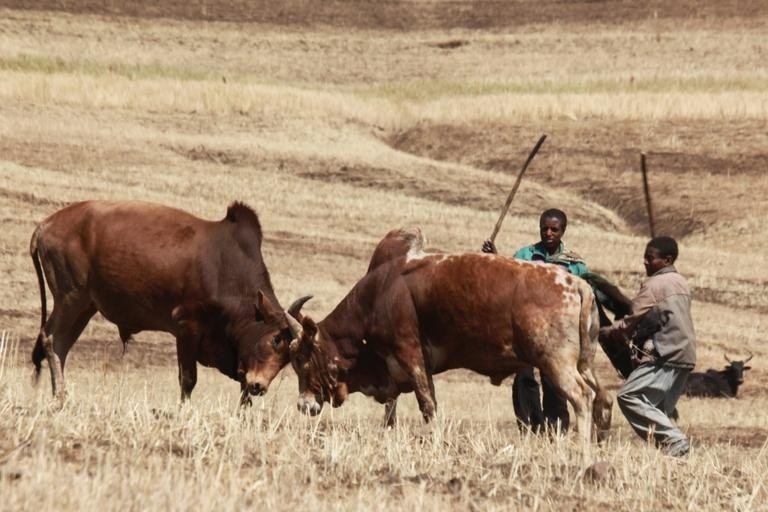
[289,227,613,455]
[30,201,300,413]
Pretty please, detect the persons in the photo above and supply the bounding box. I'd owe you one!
[481,209,591,444]
[597,236,697,459]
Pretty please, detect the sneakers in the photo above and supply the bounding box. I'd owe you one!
[662,439,691,455]
